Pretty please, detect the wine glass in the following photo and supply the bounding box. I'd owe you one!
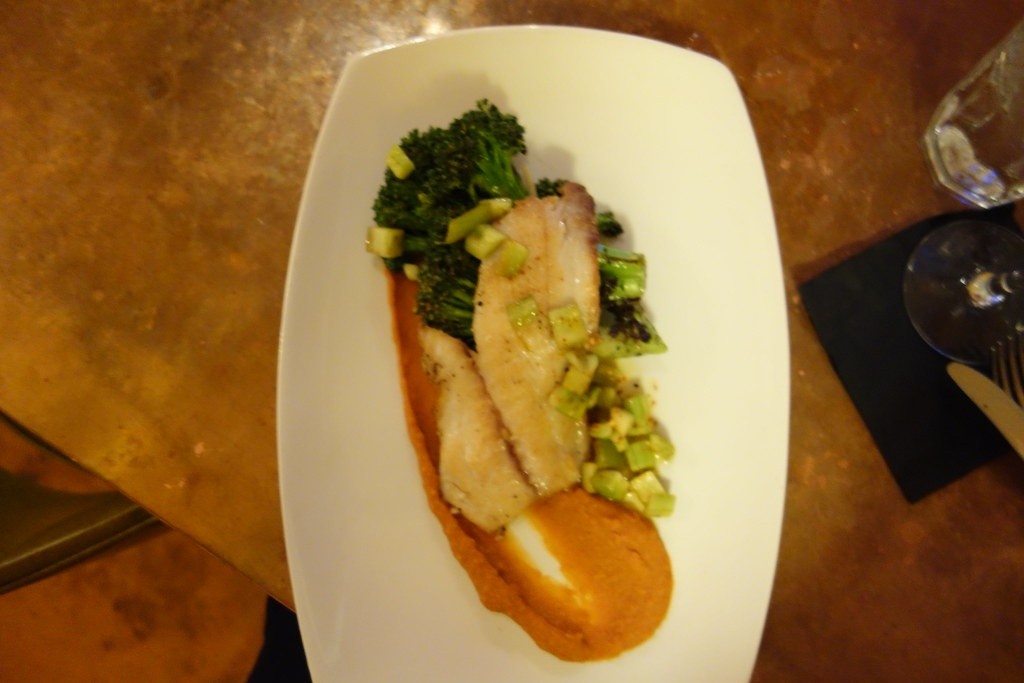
[904,220,1023,362]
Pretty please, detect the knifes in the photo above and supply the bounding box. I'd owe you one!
[946,362,1024,461]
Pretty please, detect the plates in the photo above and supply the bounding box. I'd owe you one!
[275,22,791,683]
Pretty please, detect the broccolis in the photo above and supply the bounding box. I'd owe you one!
[370,104,666,358]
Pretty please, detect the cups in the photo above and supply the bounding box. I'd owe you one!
[922,24,1024,210]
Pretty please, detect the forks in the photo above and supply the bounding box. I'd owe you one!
[991,348,1024,408]
